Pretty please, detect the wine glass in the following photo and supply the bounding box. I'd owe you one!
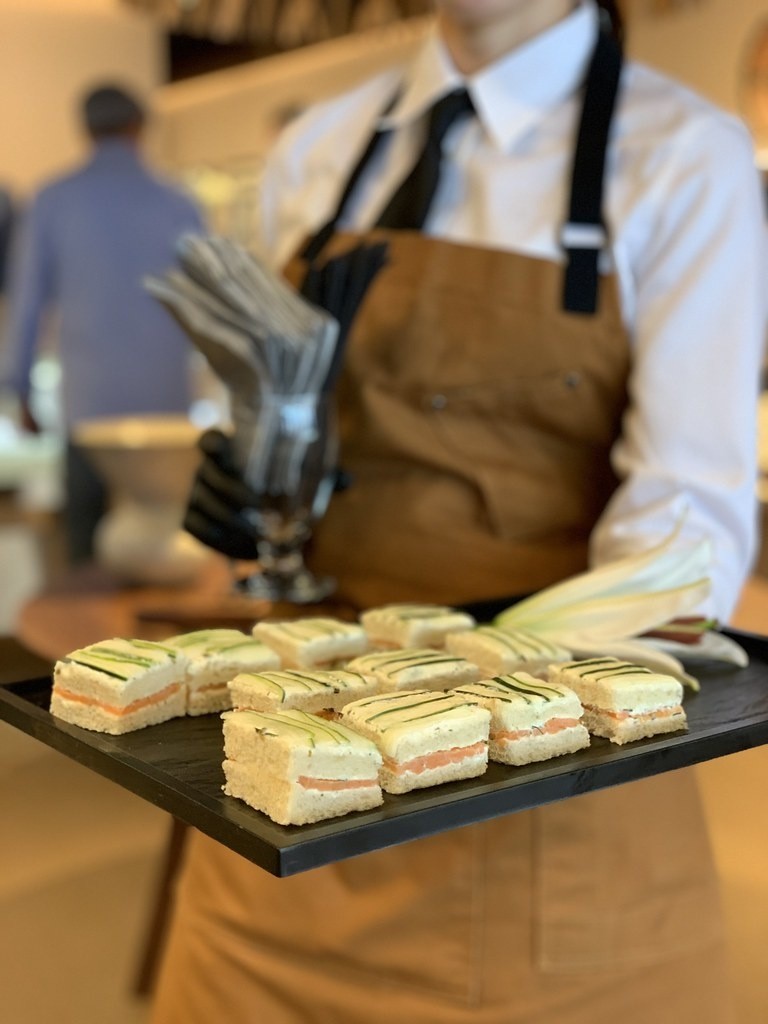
[233,388,340,603]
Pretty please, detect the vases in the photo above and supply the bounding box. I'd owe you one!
[78,416,218,578]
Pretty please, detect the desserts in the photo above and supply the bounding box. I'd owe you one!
[48,603,688,828]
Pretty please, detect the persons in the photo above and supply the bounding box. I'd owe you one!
[0,86,207,581]
[157,0,768,1024]
